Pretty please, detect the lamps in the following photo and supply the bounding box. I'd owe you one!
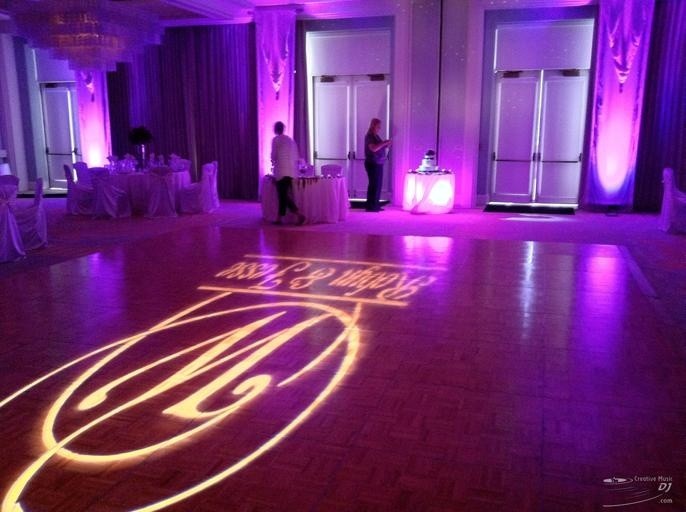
[6,1,169,92]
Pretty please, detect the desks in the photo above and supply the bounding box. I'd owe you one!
[258,174,350,226]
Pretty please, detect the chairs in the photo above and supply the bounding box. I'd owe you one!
[319,163,345,174]
[0,153,220,265]
[657,167,685,236]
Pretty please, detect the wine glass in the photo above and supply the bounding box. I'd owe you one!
[298,164,308,175]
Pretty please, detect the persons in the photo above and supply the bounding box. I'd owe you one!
[270,120,308,227]
[363,118,392,213]
